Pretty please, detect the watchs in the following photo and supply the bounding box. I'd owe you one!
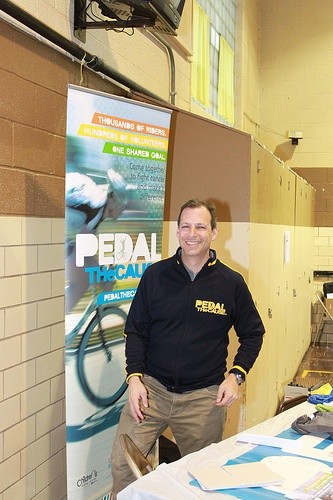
[228,368,245,385]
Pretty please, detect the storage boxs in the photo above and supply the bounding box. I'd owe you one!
[283,375,322,402]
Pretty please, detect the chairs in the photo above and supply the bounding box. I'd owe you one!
[119,433,153,479]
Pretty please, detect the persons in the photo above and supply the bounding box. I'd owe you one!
[66,168,131,314]
[112,198,265,499]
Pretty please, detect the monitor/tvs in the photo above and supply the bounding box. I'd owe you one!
[150,0,185,29]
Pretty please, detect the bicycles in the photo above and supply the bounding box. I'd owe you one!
[65,265,132,408]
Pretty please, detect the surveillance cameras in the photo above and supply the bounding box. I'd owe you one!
[288,131,303,144]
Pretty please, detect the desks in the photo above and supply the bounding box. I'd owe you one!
[115,402,333,499]
